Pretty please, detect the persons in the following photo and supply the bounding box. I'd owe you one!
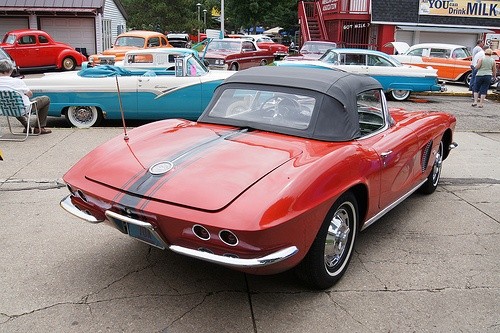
[472,45,489,102]
[472,49,496,108]
[0,57,52,134]
[468,39,485,100]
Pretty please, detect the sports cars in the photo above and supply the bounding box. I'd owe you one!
[58,66,457,291]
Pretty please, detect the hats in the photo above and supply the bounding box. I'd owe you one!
[485,49,494,54]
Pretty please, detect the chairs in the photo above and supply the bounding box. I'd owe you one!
[142,70,157,77]
[0,85,41,142]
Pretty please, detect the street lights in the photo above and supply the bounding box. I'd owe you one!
[202,8,208,34]
[196,2,202,43]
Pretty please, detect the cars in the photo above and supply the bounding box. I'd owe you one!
[88,30,175,70]
[26,48,239,129]
[224,35,290,61]
[273,48,441,102]
[198,38,276,71]
[281,41,336,62]
[0,29,87,71]
[166,23,295,52]
[373,41,500,88]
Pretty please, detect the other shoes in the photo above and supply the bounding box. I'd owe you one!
[479,105,483,107]
[23,127,32,133]
[472,104,477,106]
[34,127,51,134]
